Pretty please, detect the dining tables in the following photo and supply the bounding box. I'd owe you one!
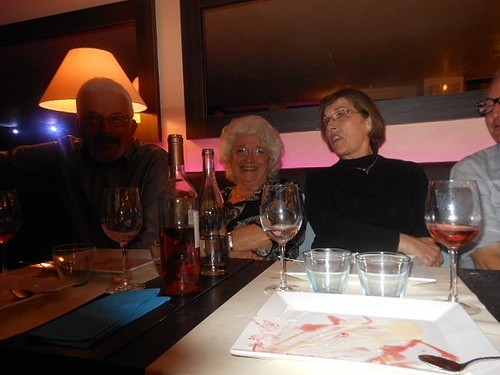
[0,223,500,375]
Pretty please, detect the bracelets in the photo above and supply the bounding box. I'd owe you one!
[227,232,233,251]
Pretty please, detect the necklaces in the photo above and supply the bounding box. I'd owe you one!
[356,155,379,174]
[235,189,255,199]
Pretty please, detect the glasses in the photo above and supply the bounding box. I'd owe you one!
[320,109,357,129]
[475,97,500,115]
[79,114,133,128]
[232,147,269,160]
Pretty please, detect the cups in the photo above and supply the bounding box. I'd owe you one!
[303,248,351,293]
[150,241,161,273]
[52,243,96,286]
[357,253,410,299]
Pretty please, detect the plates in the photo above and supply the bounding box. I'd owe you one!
[230,290,500,375]
[286,253,437,286]
[29,249,161,274]
[0,273,76,311]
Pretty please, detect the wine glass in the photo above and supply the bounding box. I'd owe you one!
[101,185,143,294]
[424,178,483,315]
[259,184,302,294]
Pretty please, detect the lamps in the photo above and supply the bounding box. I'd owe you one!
[38,47,147,117]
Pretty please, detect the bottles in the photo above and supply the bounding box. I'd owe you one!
[200,149,229,276]
[157,134,201,295]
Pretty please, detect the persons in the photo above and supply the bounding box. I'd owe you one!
[196,115,308,261]
[448,73,500,271]
[304,87,444,267]
[0,78,169,270]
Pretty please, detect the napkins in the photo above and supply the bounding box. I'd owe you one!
[30,288,171,349]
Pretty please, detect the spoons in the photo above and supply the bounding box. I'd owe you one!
[10,287,62,298]
[417,354,500,373]
